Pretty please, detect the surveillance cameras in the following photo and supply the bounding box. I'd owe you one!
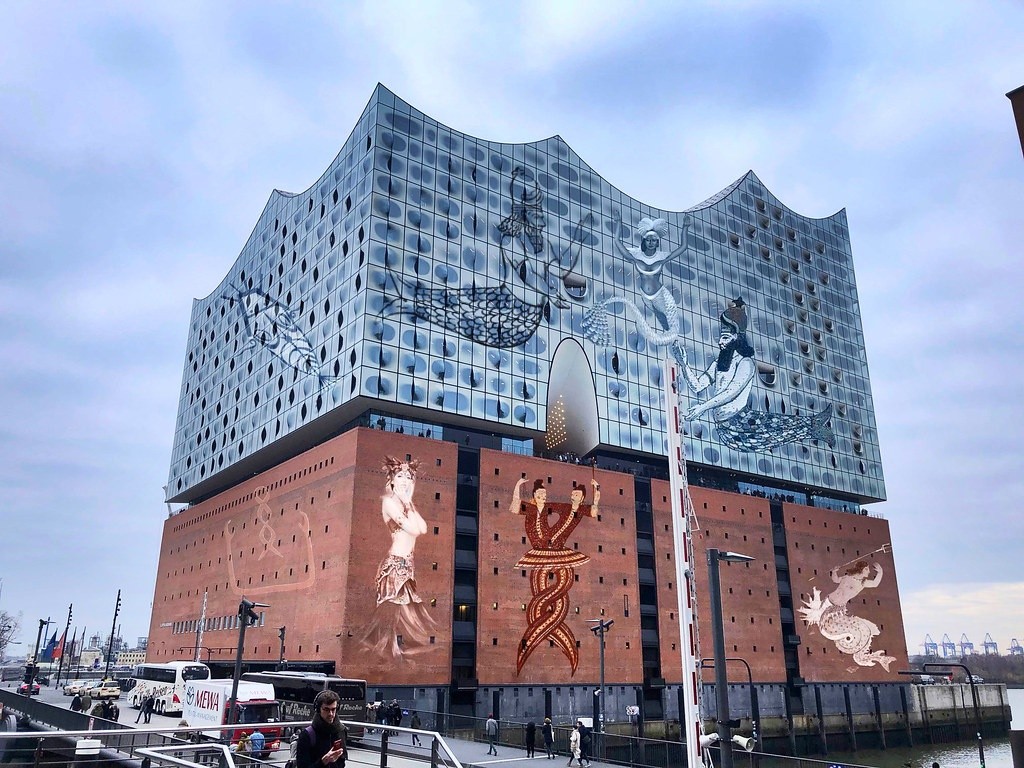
[603,619,614,627]
[248,610,260,620]
[591,625,601,631]
[39,619,46,625]
[241,600,254,609]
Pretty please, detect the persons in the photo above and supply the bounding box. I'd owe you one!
[70,693,264,761]
[367,698,592,768]
[296,690,348,768]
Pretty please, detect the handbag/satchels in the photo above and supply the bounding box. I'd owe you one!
[581,734,591,745]
[284,757,297,768]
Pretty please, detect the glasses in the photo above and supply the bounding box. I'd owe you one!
[321,706,337,713]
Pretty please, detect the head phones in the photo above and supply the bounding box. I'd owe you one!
[314,689,341,714]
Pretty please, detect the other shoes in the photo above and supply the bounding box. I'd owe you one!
[418,742,421,746]
[487,752,491,755]
[494,752,497,756]
[365,727,399,736]
[585,763,591,767]
[547,755,555,759]
[567,762,570,766]
[527,755,534,758]
[579,763,583,768]
[577,762,581,766]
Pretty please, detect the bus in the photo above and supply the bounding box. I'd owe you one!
[242,670,366,745]
[123,660,211,716]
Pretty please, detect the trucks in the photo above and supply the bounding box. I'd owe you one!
[182,678,282,760]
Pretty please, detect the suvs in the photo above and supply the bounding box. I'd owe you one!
[965,675,985,684]
[89,681,121,700]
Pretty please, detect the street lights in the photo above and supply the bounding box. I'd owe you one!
[705,547,755,768]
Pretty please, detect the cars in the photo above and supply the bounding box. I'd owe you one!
[71,665,130,671]
[913,675,935,685]
[17,681,40,695]
[63,681,85,696]
[79,682,98,697]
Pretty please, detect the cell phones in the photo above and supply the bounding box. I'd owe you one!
[333,739,342,750]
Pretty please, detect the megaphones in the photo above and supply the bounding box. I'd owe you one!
[732,734,755,752]
[699,732,719,748]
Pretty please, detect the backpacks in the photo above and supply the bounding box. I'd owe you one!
[243,741,252,754]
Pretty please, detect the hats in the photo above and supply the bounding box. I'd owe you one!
[545,718,551,724]
[293,726,301,733]
[487,713,493,718]
